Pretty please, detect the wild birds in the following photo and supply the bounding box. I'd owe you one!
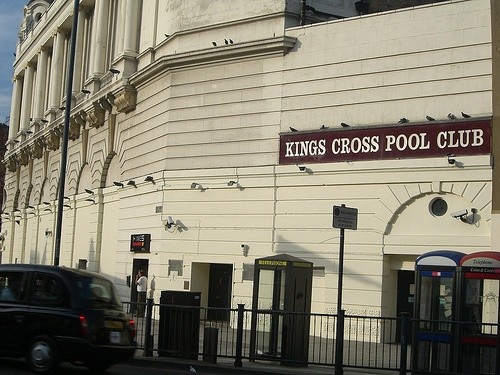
[461,112,471,118]
[224,38,228,45]
[341,123,350,128]
[229,38,233,44]
[426,116,435,121]
[211,41,216,46]
[289,127,297,132]
[398,118,406,123]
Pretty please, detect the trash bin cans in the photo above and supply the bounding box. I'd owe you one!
[157,290,202,360]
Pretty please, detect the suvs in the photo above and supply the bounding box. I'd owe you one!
[0,264,137,375]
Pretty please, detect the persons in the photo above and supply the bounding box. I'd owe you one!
[1,274,18,301]
[134,269,147,318]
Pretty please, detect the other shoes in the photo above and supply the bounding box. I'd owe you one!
[133,311,145,318]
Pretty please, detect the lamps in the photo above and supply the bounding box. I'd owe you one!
[127,181,137,188]
[448,154,455,164]
[297,163,305,172]
[228,181,241,189]
[191,183,208,190]
[144,176,155,184]
